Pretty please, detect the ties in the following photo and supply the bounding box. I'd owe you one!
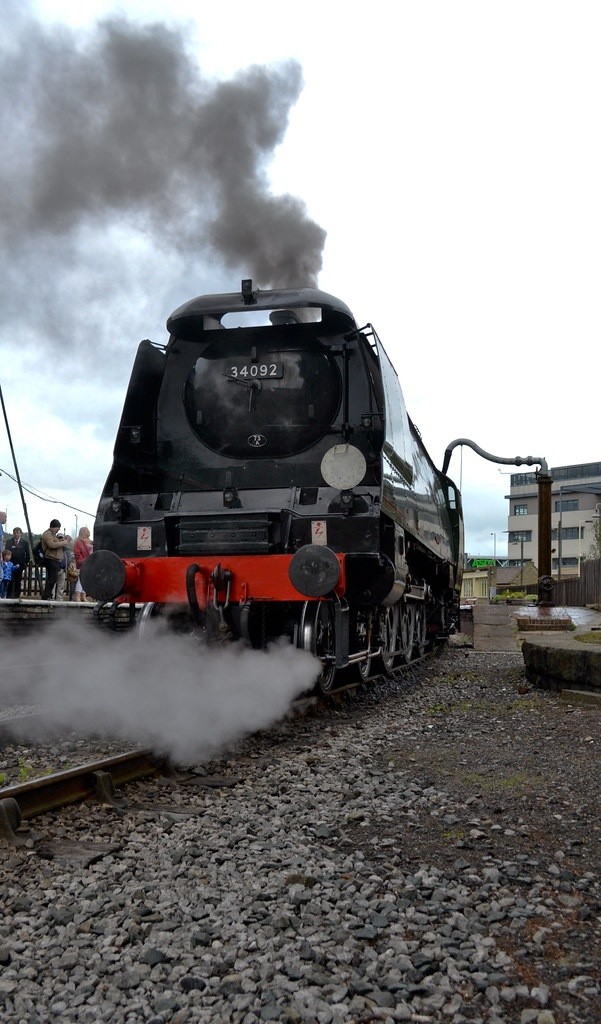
[15,541,18,545]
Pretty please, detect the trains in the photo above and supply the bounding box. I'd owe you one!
[79,279,466,698]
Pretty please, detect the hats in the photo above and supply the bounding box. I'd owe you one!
[57,533,64,538]
[50,519,61,527]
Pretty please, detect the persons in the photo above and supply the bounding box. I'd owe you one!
[0,511,93,602]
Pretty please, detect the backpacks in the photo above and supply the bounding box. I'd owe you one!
[33,538,49,566]
